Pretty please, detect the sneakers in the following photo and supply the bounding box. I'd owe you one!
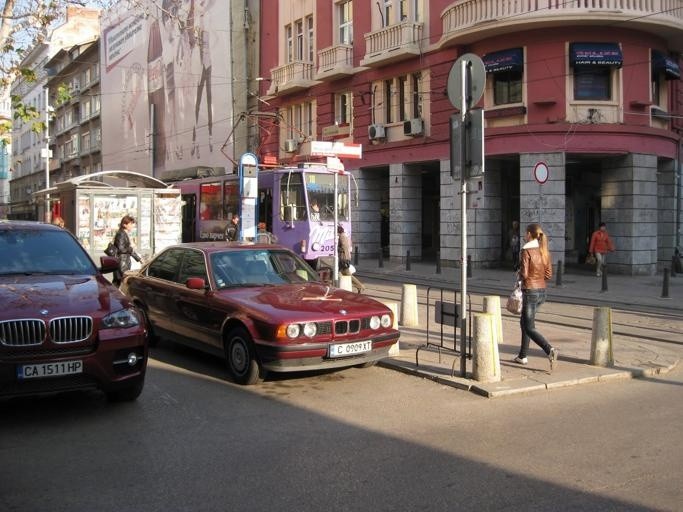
[512,357,528,365]
[548,348,559,375]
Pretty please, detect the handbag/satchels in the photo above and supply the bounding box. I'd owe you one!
[104,243,118,258]
[339,259,351,270]
[585,256,597,266]
[506,288,524,315]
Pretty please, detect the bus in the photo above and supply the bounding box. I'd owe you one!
[164,161,359,263]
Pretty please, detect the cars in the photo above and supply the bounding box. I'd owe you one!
[118,240,400,387]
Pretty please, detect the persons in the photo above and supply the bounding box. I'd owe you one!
[222,214,241,241]
[334,223,366,295]
[507,219,520,268]
[119,64,142,139]
[110,215,146,288]
[188,13,216,154]
[589,222,616,277]
[161,1,185,159]
[510,223,559,372]
[256,220,279,245]
[304,197,323,220]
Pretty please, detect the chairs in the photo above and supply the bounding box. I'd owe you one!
[246,260,271,285]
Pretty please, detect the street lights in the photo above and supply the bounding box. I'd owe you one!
[42,83,54,224]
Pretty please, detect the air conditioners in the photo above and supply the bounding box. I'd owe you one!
[366,123,385,140]
[401,117,423,137]
[282,139,298,153]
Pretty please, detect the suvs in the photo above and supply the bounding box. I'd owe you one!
[0,218,148,406]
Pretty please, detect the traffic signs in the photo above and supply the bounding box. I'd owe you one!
[309,140,363,159]
[325,156,345,174]
